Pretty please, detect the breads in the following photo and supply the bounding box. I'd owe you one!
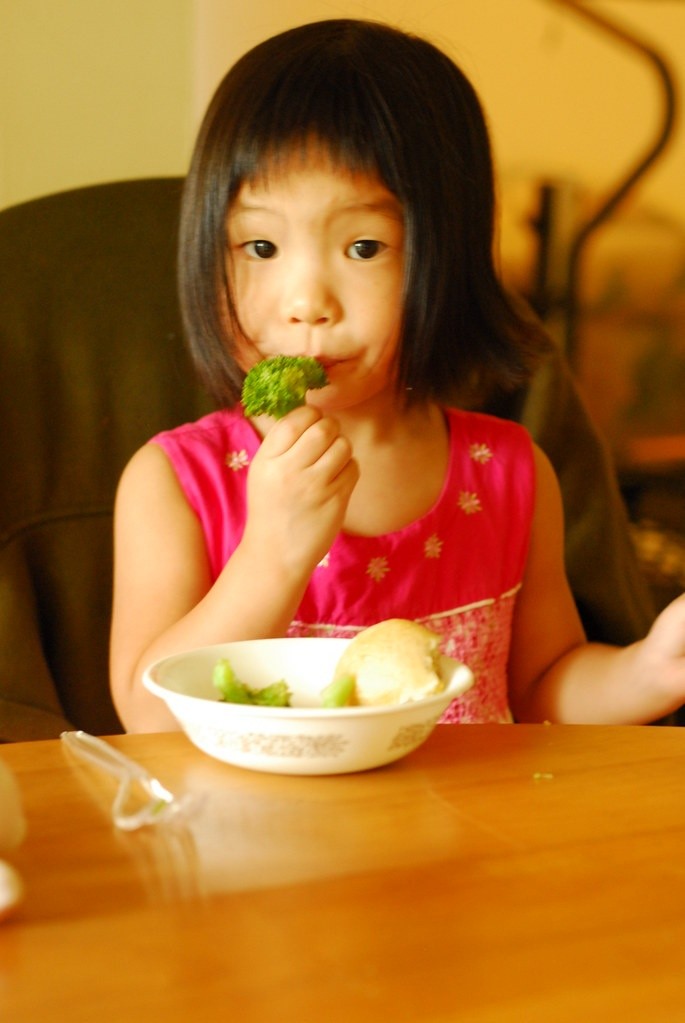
[334,618,443,707]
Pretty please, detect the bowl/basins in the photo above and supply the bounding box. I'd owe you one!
[143,638,474,775]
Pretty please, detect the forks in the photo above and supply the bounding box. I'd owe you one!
[61,732,207,831]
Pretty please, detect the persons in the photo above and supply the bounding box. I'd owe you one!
[108,19,685,735]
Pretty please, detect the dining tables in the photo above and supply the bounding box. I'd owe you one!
[0,723,684,1022]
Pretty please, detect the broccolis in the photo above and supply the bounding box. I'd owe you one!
[211,657,355,709]
[240,355,329,422]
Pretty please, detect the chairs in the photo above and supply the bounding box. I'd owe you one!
[1,173,684,744]
